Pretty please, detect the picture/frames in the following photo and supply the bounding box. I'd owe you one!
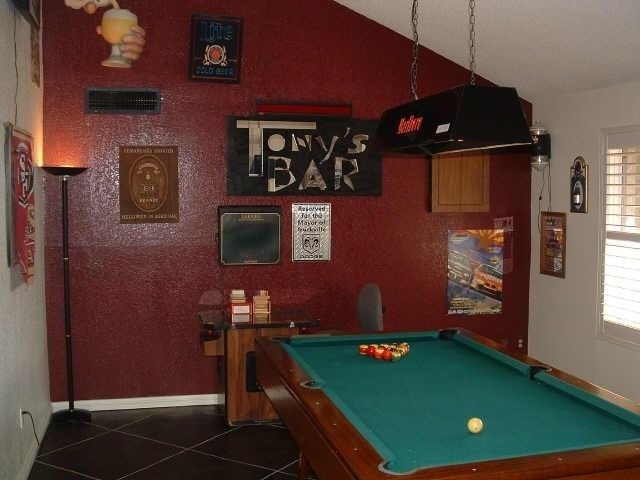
[5,123,36,270]
[189,14,242,84]
[539,210,566,280]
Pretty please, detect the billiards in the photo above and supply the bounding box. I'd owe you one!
[467,417,483,434]
[359,342,410,363]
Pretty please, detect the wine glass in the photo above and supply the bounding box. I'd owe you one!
[100,8,138,69]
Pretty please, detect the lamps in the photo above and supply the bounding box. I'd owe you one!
[368,0,534,158]
[529,120,551,174]
[41,166,92,424]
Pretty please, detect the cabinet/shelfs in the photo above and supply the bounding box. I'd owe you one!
[199,322,297,426]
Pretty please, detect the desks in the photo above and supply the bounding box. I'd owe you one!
[255,324,640,480]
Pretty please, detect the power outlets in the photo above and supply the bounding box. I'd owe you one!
[19,407,33,431]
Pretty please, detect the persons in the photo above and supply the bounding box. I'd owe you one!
[95,20,147,61]
